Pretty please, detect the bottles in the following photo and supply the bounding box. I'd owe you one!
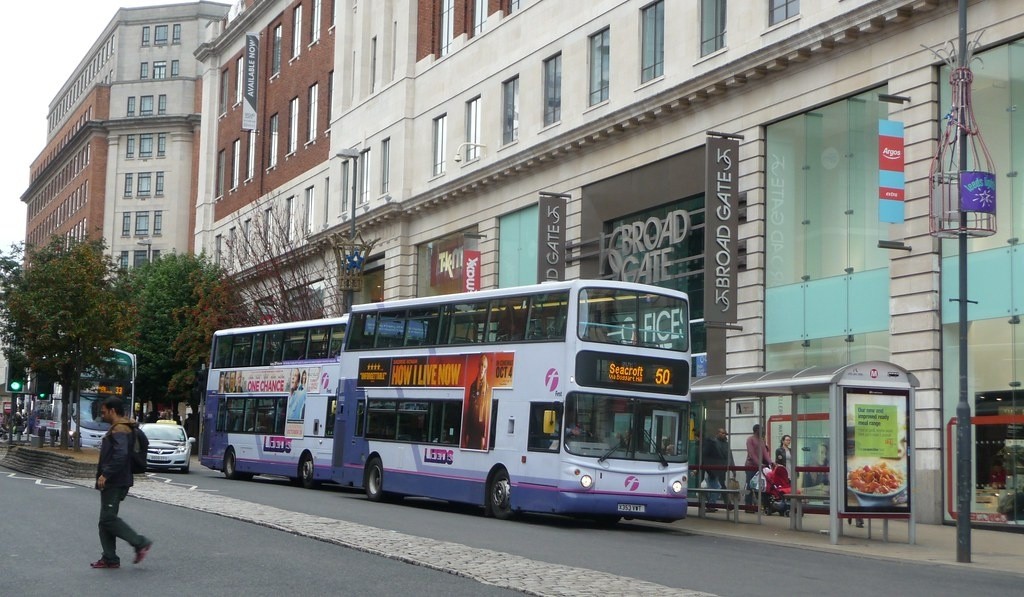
[508,416,515,435]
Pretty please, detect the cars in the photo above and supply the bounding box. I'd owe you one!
[137,419,196,476]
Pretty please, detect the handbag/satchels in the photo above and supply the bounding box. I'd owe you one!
[749,471,767,492]
[729,477,739,490]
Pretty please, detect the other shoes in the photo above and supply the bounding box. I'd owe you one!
[725,504,734,509]
[133,542,151,564]
[90,558,121,568]
[745,510,755,513]
[705,507,719,512]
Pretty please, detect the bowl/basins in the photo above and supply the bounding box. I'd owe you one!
[846,473,908,506]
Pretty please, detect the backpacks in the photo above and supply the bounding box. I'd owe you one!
[120,422,149,474]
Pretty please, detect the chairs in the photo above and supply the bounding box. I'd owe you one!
[359,334,519,348]
[221,349,281,368]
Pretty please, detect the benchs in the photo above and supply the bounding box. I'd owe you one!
[688,488,740,523]
[784,494,830,531]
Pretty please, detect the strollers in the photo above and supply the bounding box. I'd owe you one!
[762,464,805,518]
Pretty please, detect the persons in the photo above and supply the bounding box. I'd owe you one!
[136,409,200,435]
[802,444,830,488]
[463,354,491,451]
[287,367,306,419]
[744,425,772,513]
[0,411,34,441]
[775,434,792,484]
[550,406,593,439]
[89,395,153,568]
[219,371,243,393]
[690,428,736,513]
[990,461,1006,489]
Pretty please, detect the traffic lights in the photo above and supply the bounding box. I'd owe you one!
[5,360,25,393]
[35,391,51,403]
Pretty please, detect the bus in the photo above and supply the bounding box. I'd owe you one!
[24,347,138,452]
[330,278,696,529]
[199,312,350,491]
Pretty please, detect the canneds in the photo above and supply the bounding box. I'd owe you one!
[819,530,828,534]
